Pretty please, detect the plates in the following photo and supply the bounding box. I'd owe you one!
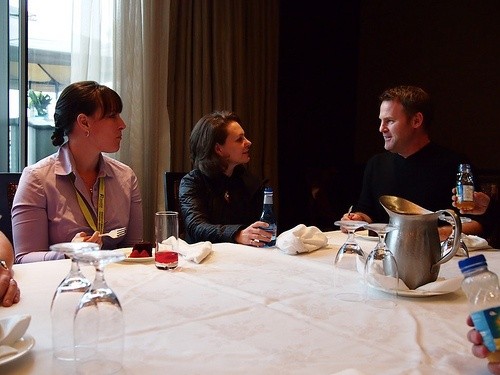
[0,325,35,367]
[360,253,464,298]
[112,247,160,261]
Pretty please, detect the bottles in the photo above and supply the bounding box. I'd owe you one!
[154,211,179,270]
[457,163,474,211]
[256,187,277,248]
[457,254,500,365]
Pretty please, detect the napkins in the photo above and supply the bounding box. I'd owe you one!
[0,315,31,357]
[275,224,328,256]
[162,234,213,264]
[443,230,488,256]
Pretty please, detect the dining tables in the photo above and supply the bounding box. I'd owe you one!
[0,228,500,375]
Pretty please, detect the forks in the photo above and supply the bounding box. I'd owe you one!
[96,226,128,239]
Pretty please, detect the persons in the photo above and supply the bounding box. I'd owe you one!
[467,315,500,375]
[452,187,500,250]
[0,231,20,307]
[179,112,272,248]
[11,83,145,264]
[341,86,482,241]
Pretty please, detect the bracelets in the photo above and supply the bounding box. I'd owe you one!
[0,262,8,271]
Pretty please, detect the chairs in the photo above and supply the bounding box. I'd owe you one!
[470,168,500,249]
[0,171,191,241]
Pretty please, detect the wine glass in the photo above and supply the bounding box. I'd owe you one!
[48,242,102,361]
[72,246,126,375]
[332,219,368,301]
[362,222,399,307]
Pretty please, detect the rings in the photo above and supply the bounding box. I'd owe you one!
[251,241,253,244]
[10,279,16,285]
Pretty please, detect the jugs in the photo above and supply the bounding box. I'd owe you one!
[376,192,462,291]
